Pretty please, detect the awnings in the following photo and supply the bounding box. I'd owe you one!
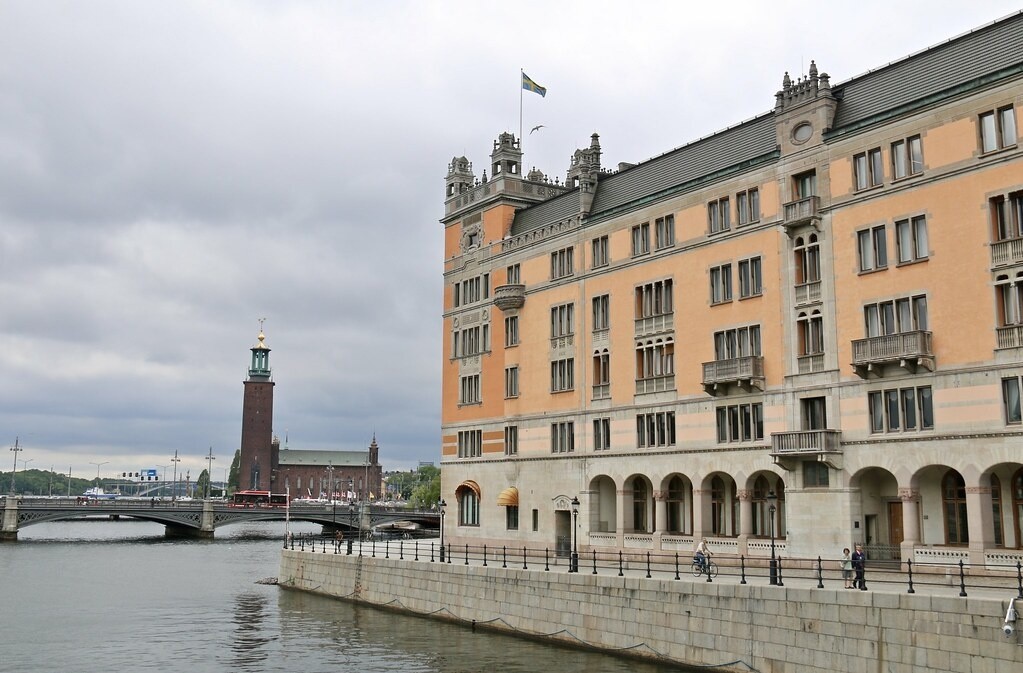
[497,487,517,506]
[455,480,480,502]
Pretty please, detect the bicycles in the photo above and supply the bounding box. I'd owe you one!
[691,555,718,578]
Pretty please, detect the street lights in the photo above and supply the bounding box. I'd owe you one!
[89,461,110,500]
[347,501,355,555]
[570,496,580,572]
[18,458,34,494]
[439,498,447,562]
[331,480,354,545]
[157,464,174,497]
[766,489,778,585]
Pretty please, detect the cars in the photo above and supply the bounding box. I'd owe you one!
[290,498,409,508]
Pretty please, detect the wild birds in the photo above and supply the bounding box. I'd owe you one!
[529,125,546,135]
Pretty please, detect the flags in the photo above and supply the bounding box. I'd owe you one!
[523,72,546,97]
[323,491,375,498]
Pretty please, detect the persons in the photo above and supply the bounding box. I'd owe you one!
[840,545,854,589]
[76,496,98,505]
[852,545,865,588]
[696,538,712,572]
[151,496,154,507]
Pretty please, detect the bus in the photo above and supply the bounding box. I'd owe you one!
[228,489,290,510]
